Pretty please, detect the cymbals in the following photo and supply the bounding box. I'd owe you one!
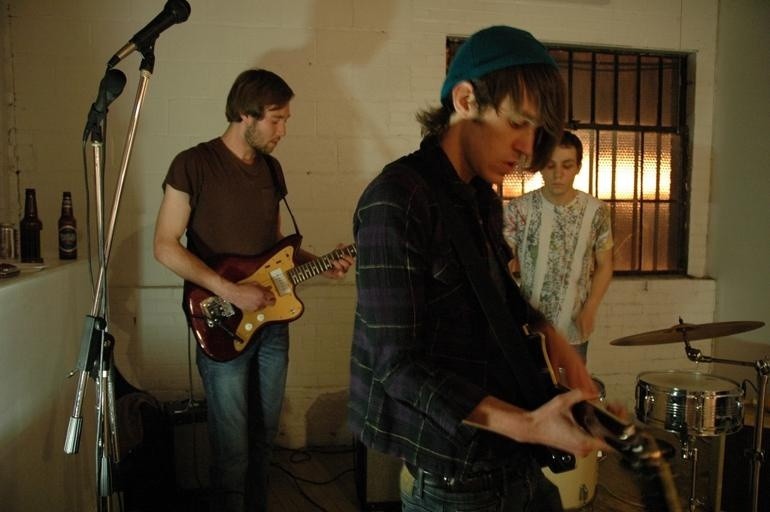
[609,315,766,346]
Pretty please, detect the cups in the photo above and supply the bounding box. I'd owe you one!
[1,222,17,261]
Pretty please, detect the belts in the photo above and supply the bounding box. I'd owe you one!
[403,460,502,492]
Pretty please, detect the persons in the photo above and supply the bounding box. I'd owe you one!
[152,65,355,510]
[499,130,616,372]
[352,23,628,508]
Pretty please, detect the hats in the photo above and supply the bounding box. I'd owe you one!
[441,26,560,106]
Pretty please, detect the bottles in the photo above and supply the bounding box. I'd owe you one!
[56,191,78,261]
[19,188,44,265]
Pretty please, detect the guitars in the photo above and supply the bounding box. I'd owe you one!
[522,324,679,475]
[190,233,357,363]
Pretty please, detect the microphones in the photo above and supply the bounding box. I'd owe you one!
[107,0,191,69]
[82,69,127,142]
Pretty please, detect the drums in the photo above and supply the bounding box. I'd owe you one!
[635,370,746,438]
[541,364,608,509]
